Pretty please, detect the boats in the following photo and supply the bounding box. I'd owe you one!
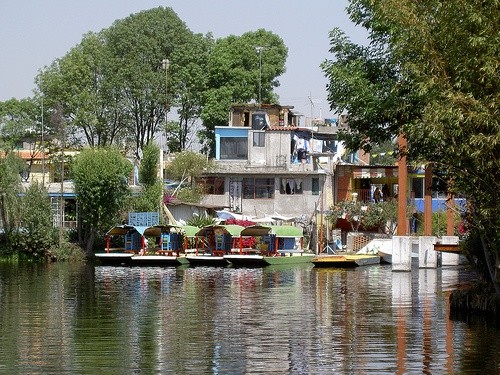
[91,221,245,266]
[375,246,471,265]
[309,252,381,267]
[223,224,319,266]
